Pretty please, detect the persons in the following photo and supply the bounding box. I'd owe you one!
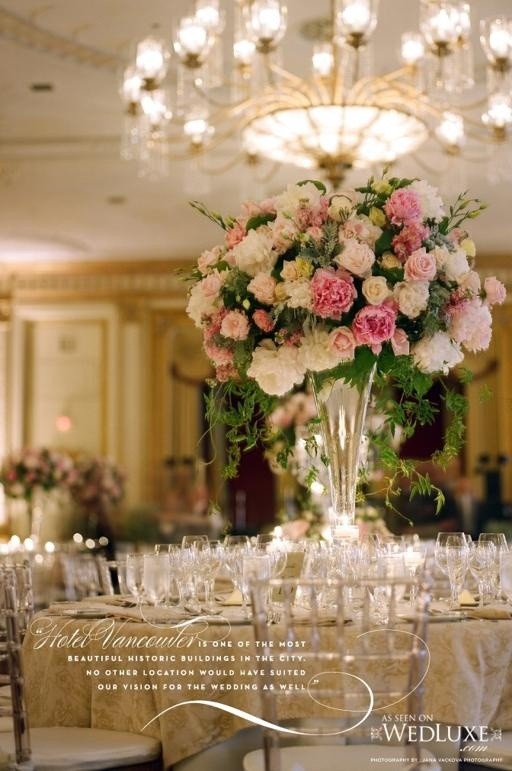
[151,453,221,545]
[363,451,510,540]
[61,482,120,593]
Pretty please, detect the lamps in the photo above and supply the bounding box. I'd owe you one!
[114,0,512,193]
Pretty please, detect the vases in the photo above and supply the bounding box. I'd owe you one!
[307,361,378,544]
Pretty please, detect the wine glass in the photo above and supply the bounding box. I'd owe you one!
[124,532,510,626]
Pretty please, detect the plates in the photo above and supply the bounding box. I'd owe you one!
[62,605,124,620]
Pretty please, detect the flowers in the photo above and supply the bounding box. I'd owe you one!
[0,442,128,517]
[175,159,510,541]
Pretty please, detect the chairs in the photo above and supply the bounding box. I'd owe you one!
[0,551,442,771]
